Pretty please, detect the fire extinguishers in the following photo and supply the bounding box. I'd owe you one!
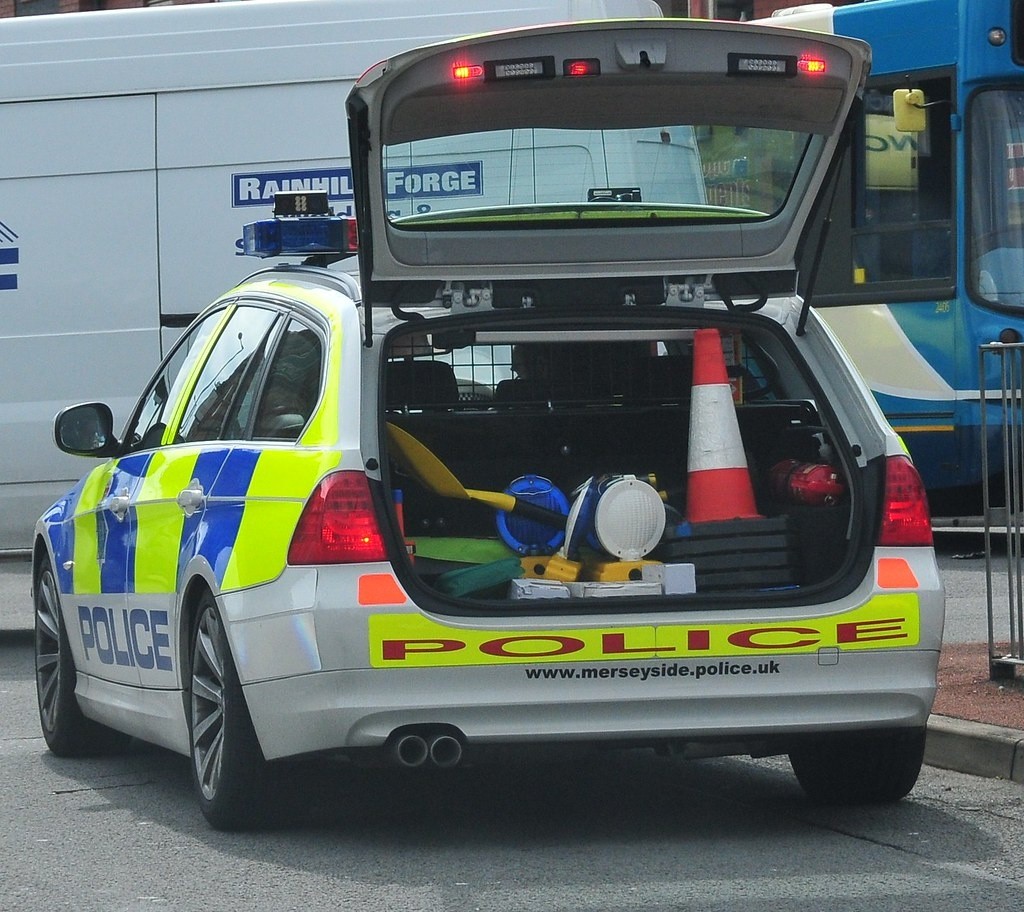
[766,457,848,507]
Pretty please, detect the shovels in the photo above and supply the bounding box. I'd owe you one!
[378,420,572,527]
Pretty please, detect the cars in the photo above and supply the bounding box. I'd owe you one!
[29,19,948,833]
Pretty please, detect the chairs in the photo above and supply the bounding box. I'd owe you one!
[384,360,544,413]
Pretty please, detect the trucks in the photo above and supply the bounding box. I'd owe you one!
[0,0,710,631]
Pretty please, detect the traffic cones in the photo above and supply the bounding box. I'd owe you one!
[662,328,798,590]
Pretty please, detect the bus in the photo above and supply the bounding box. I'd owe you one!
[698,1,1024,533]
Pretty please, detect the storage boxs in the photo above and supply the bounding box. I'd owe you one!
[508,563,696,600]
